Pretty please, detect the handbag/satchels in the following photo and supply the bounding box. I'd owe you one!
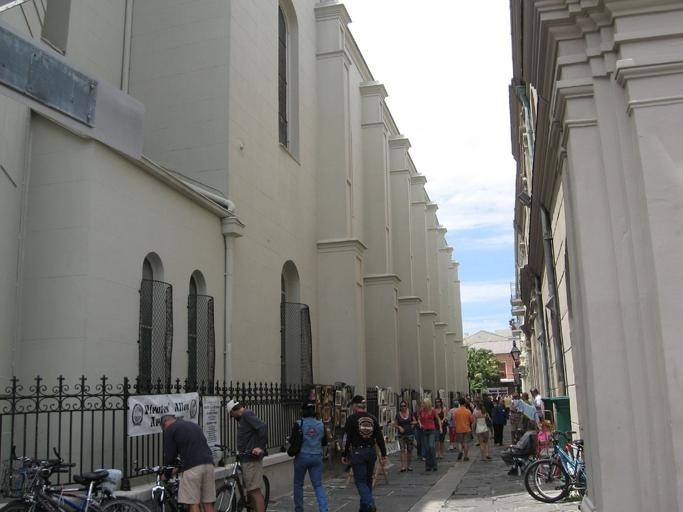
[287,430,304,457]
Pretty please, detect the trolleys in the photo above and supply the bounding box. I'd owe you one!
[532,410,557,457]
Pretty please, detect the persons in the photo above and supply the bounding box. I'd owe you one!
[339,395,388,511]
[392,388,553,475]
[159,409,216,511]
[285,401,330,512]
[226,399,267,512]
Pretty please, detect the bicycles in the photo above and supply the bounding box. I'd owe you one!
[0,445,151,511]
[132,457,189,512]
[524,430,586,502]
[209,443,269,511]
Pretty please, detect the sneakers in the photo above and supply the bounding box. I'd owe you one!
[426,467,440,471]
[458,452,463,460]
[447,441,504,451]
[400,467,413,472]
[464,457,469,460]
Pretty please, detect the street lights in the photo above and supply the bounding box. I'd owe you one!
[511,341,521,397]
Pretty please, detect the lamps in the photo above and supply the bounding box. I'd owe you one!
[509,336,521,362]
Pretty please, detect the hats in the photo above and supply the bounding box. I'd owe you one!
[349,395,366,404]
[225,400,239,413]
[301,401,315,410]
